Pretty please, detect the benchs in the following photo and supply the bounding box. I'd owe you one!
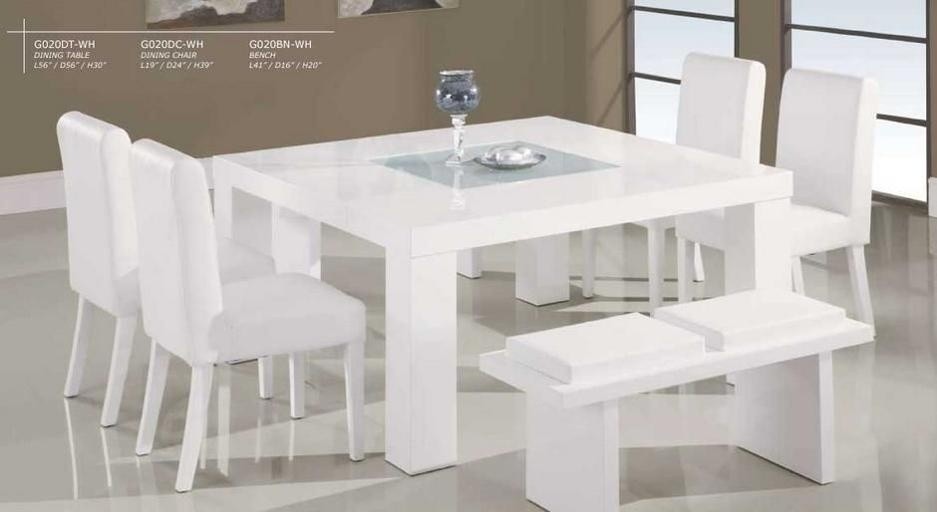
[477,285,876,512]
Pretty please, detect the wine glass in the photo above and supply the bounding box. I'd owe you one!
[435,69,482,168]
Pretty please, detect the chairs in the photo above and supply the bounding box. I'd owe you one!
[55,109,275,429]
[130,135,370,494]
[580,50,769,302]
[675,67,878,343]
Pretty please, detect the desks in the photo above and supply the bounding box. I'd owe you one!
[208,110,797,481]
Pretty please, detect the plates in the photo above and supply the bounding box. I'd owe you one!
[476,153,547,171]
